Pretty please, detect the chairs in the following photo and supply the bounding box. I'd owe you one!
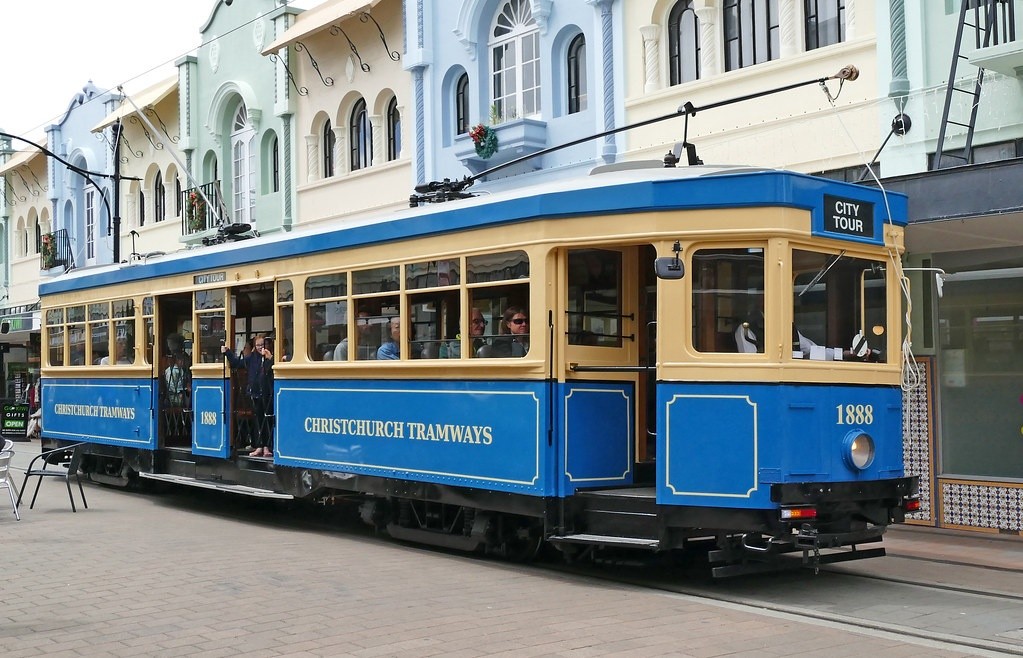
[318,334,494,362]
[74,353,173,413]
[0,440,90,520]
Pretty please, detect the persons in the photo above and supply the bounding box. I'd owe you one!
[576,330,597,345]
[70,330,191,407]
[333,308,378,360]
[377,317,423,359]
[220,333,291,456]
[491,306,528,357]
[450,308,485,358]
[735,281,872,361]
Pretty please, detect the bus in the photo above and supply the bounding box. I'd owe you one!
[38,64,947,580]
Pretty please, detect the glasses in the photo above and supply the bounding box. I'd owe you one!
[509,319,529,324]
[256,343,264,347]
[471,319,488,325]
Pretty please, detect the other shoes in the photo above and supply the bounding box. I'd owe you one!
[262,447,272,457]
[249,448,263,456]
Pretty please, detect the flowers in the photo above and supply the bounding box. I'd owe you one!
[470,123,498,158]
